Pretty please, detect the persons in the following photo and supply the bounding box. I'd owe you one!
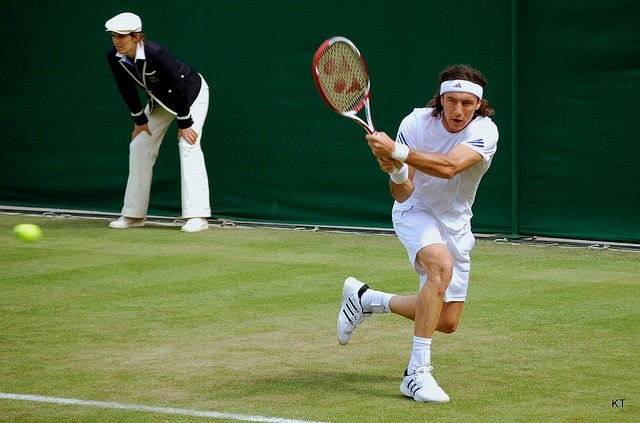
[104,12,212,233]
[336,63,500,404]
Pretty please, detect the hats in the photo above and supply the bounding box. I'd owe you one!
[104,12,142,35]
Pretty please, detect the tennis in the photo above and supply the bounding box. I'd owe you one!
[12,222,43,244]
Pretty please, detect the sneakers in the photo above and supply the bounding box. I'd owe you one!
[399,364,450,404]
[109,216,145,229]
[181,216,209,232]
[336,276,373,345]
